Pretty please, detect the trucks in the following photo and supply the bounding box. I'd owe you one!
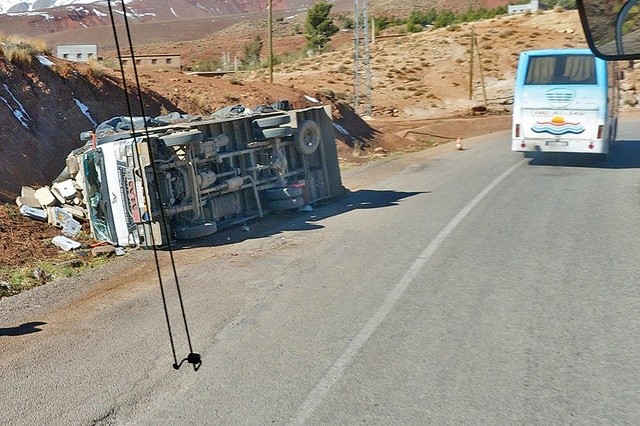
[67,103,343,248]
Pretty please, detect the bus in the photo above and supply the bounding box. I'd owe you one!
[512,47,619,157]
